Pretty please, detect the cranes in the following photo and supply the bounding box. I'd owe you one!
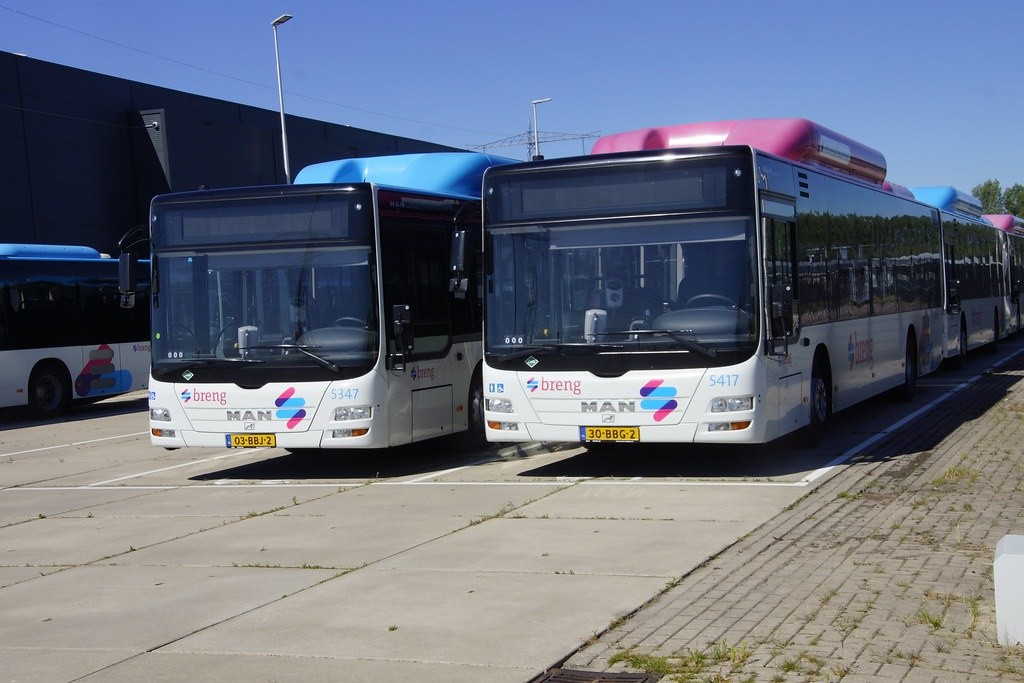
[463,118,604,162]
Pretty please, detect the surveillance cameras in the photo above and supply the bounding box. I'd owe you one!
[152,122,158,128]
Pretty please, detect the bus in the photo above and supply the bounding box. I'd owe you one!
[480,116,1023,451]
[0,240,151,419]
[117,150,533,456]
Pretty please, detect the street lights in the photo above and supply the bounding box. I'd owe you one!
[270,13,293,185]
[531,97,552,156]
